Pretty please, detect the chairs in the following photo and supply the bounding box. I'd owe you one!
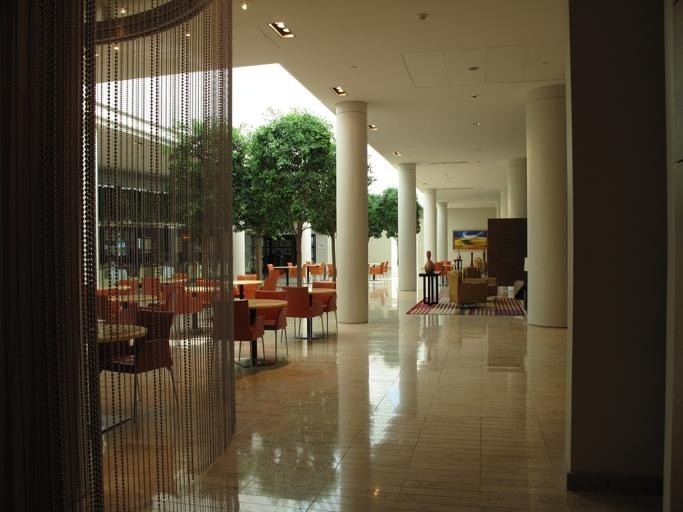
[254,290,289,361]
[210,299,266,367]
[298,281,339,335]
[98,262,222,328]
[236,262,389,291]
[433,261,497,308]
[105,310,180,425]
[280,286,325,344]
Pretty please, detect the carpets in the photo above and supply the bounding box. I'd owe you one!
[406,286,527,316]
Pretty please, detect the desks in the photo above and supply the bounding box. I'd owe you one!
[97,324,148,433]
[298,287,338,344]
[232,298,287,359]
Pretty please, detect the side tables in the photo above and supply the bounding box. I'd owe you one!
[419,271,442,306]
